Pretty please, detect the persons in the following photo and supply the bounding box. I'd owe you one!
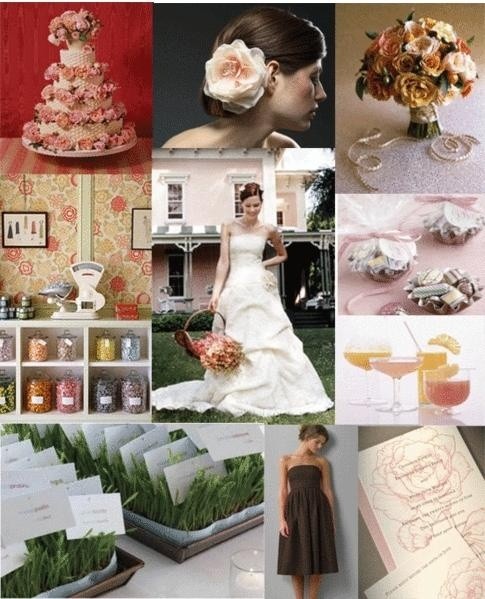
[149,180,334,418]
[162,14,327,150]
[276,423,338,599]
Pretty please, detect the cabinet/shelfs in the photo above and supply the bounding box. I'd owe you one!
[0,317,151,424]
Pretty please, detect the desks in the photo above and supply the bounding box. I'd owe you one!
[168,295,195,313]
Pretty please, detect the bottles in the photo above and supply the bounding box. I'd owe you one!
[96,330,116,361]
[23,369,52,414]
[91,369,119,413]
[54,369,82,413]
[120,330,141,361]
[0,329,14,362]
[121,369,148,414]
[0,369,16,414]
[56,330,77,361]
[0,295,35,320]
[22,330,49,362]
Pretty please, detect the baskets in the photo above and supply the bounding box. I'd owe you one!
[173,308,227,361]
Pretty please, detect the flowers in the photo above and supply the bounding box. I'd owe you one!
[353,10,480,141]
[191,332,246,380]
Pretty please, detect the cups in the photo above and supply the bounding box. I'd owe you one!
[230,549,265,598]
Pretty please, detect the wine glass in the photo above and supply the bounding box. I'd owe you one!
[344,337,471,416]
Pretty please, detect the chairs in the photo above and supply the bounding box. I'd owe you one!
[157,285,174,313]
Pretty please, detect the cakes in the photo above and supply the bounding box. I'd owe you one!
[21,10,136,154]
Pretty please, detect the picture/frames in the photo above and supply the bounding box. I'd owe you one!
[131,208,151,250]
[2,211,48,249]
